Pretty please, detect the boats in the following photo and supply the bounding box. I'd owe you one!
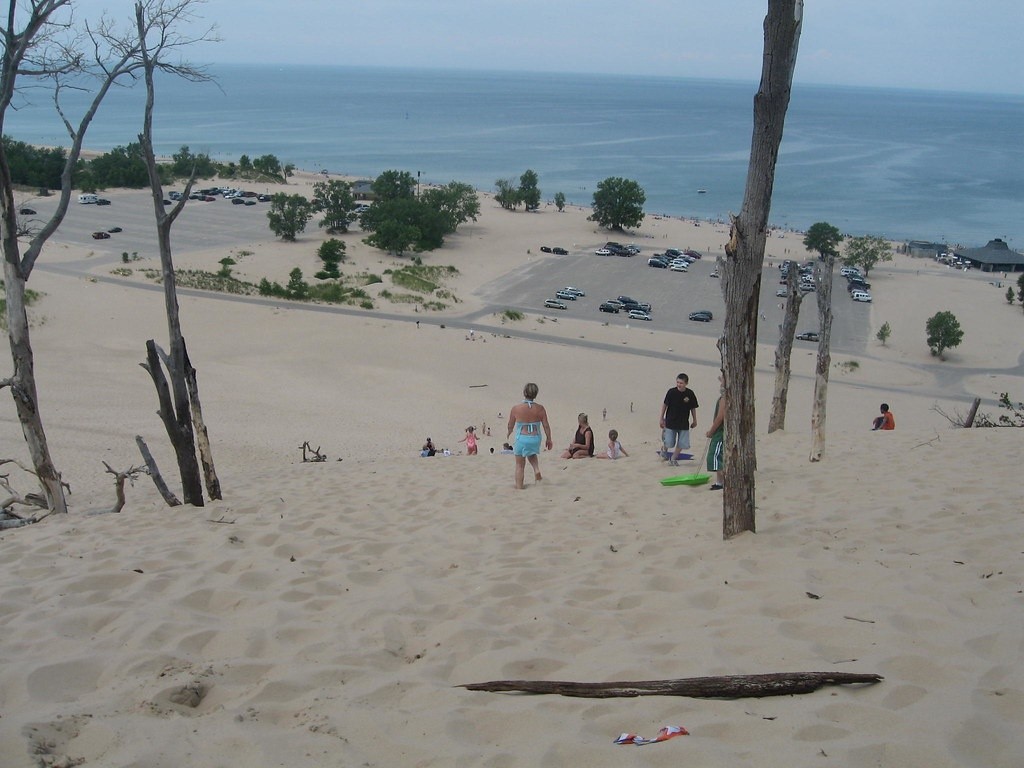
[698,189,705,193]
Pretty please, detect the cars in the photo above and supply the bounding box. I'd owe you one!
[617,296,651,315]
[648,248,702,272]
[109,227,122,233]
[628,310,652,320]
[540,246,551,252]
[840,266,872,302]
[797,332,820,341]
[565,287,585,296]
[689,314,710,322]
[351,203,371,212]
[606,299,626,310]
[95,199,111,206]
[710,266,719,278]
[596,242,640,257]
[690,311,711,319]
[556,290,576,301]
[163,188,272,205]
[544,299,567,309]
[20,209,37,215]
[599,303,619,313]
[553,248,568,255]
[93,232,110,239]
[777,259,816,297]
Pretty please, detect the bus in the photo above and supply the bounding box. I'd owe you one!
[79,194,98,204]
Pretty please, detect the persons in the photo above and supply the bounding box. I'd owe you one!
[506,383,553,488]
[481,422,486,434]
[503,443,513,450]
[498,413,503,417]
[458,426,480,456]
[704,397,725,490]
[444,449,450,457]
[596,430,629,459]
[487,428,491,436]
[423,437,435,456]
[562,413,595,458]
[873,404,895,430]
[660,373,700,466]
[420,447,430,457]
[602,408,607,419]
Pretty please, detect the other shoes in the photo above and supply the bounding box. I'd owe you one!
[710,484,723,490]
[668,458,679,466]
[660,446,668,460]
